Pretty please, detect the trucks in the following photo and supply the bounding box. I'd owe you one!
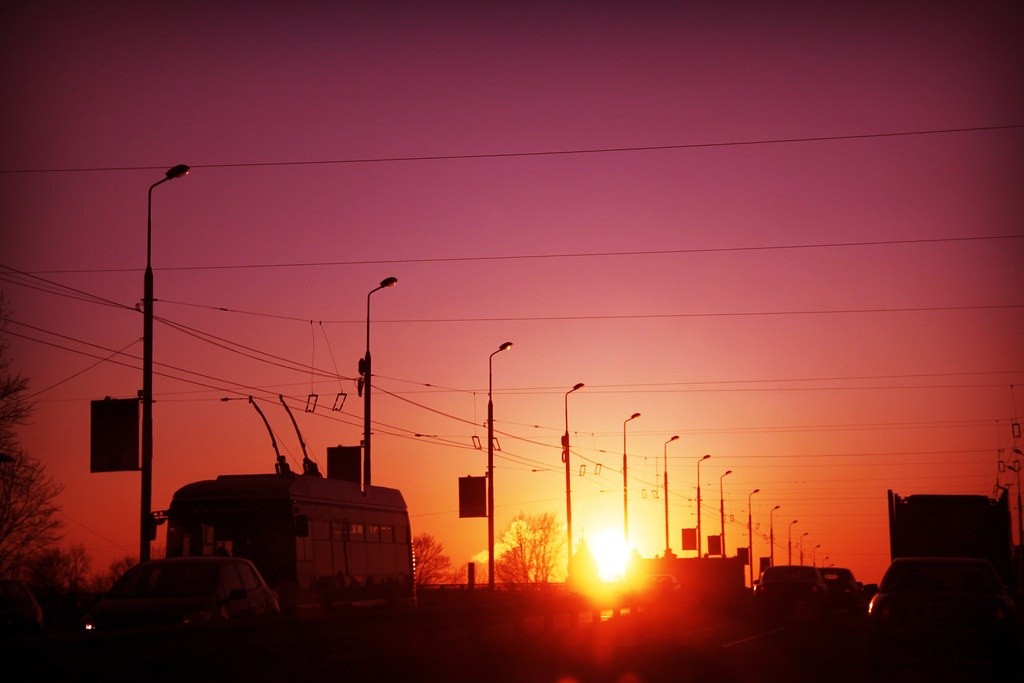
[886,489,1016,575]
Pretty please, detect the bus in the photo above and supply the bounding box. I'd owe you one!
[155,393,418,622]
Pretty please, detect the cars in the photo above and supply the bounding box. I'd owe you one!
[755,564,879,622]
[71,560,286,637]
[868,557,1013,651]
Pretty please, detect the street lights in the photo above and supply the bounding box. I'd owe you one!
[486,340,516,592]
[720,470,836,572]
[663,436,684,558]
[623,413,642,558]
[364,273,398,489]
[697,455,714,560]
[140,162,192,569]
[564,381,589,591]
